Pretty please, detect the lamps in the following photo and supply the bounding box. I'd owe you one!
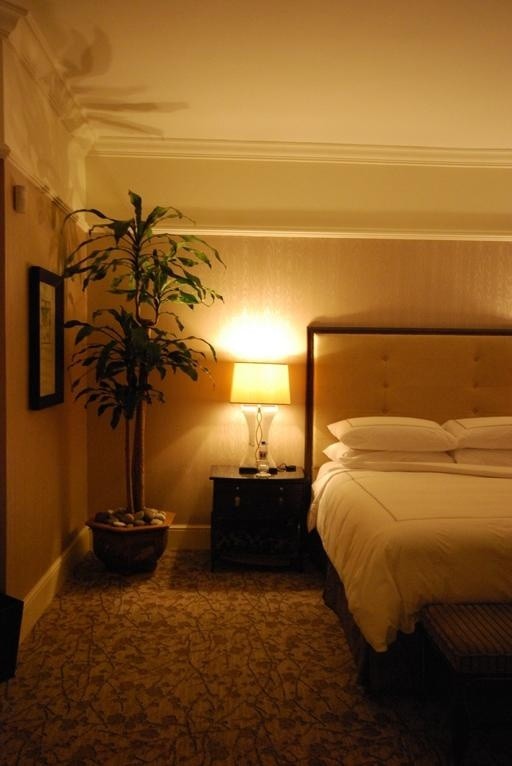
[230,361,291,474]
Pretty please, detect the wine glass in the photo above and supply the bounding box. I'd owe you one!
[256,460,271,478]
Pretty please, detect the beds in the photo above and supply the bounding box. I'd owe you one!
[305,325,512,700]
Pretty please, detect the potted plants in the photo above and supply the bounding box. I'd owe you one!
[60,190,227,574]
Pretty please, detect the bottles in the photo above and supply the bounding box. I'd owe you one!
[256,441,270,460]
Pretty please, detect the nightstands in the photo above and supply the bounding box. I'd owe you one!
[210,465,307,573]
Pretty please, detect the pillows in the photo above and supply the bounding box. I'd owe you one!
[442,416,512,449]
[326,416,458,452]
[323,441,453,469]
[450,449,512,466]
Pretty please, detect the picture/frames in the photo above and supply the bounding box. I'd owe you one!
[28,266,64,410]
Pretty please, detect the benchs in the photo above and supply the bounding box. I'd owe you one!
[417,602,512,765]
